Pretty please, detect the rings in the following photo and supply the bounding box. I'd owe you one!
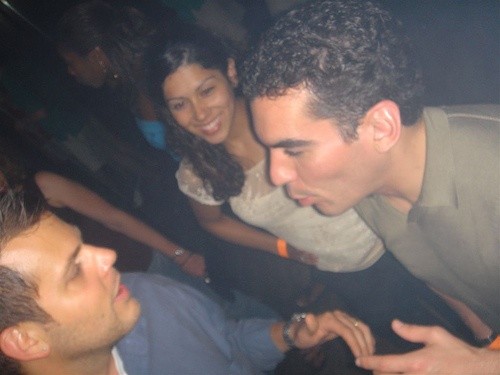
[351,317,363,329]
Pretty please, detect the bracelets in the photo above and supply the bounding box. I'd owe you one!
[474,330,497,348]
[273,237,290,258]
[179,252,195,269]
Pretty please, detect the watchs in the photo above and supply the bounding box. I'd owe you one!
[280,309,309,351]
[169,246,186,262]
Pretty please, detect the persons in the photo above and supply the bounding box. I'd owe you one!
[0,144,215,290]
[144,18,410,300]
[0,189,376,375]
[60,11,179,144]
[244,2,498,375]
